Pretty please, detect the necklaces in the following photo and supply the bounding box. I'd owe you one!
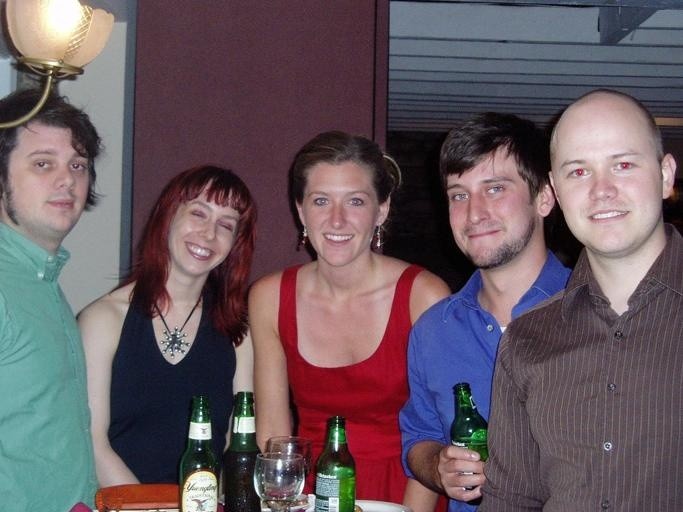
[150,284,203,358]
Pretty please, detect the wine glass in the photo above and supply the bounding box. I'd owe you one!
[264,436,305,507]
[253,451,305,512]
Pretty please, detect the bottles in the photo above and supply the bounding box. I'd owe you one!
[314,416,357,512]
[448,382,490,502]
[222,389,259,512]
[179,397,219,512]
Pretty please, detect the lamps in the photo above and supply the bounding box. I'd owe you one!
[0,1,114,128]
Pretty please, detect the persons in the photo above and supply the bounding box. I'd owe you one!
[245,132,455,510]
[401,109,577,510]
[76,163,256,493]
[477,88,683,511]
[1,88,99,512]
